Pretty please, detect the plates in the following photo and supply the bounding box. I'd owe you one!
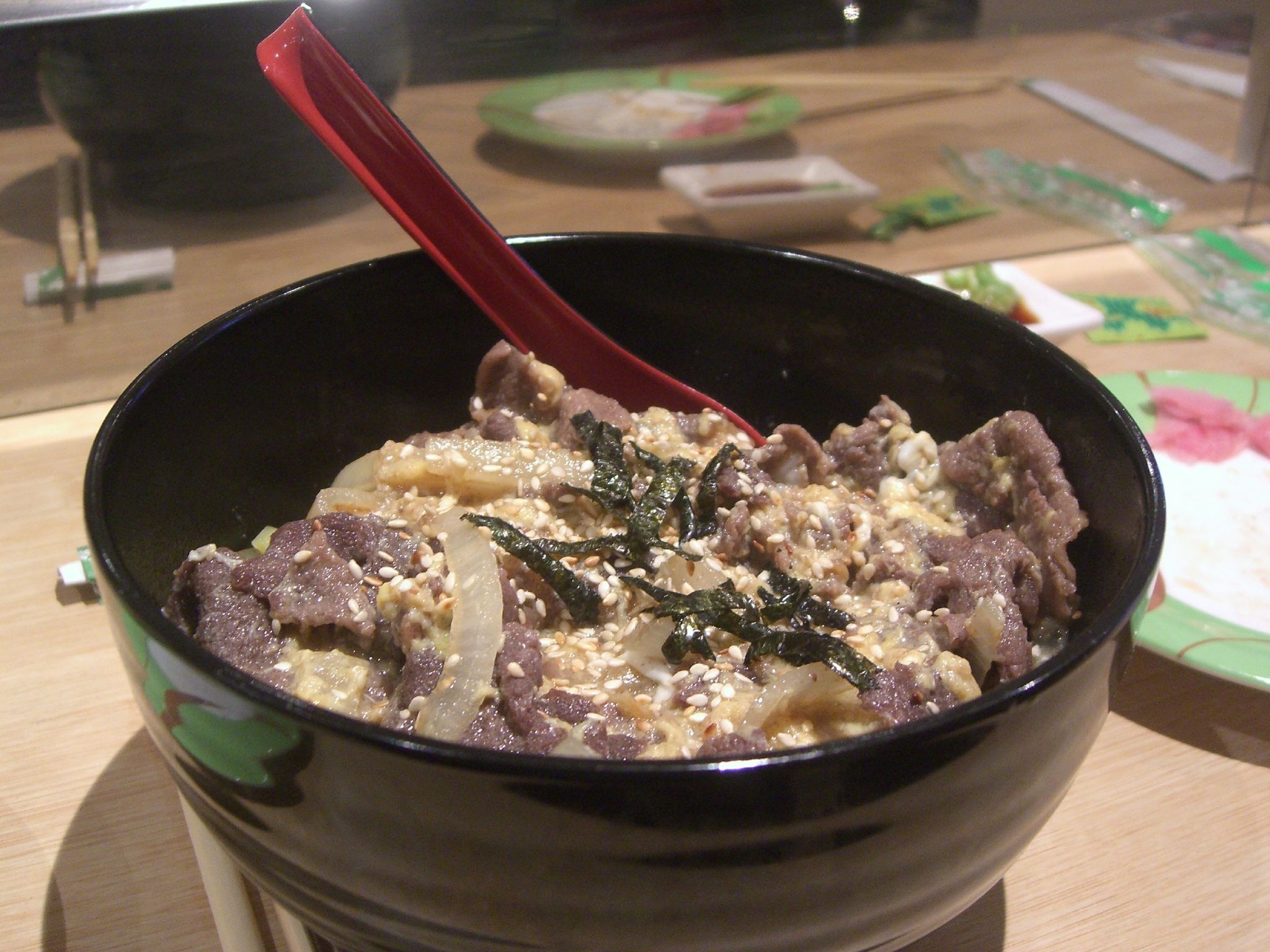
[1091,367,1270,688]
[477,65,805,163]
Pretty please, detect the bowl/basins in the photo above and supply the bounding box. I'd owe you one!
[83,229,1167,952]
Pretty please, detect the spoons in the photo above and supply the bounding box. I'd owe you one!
[254,4,767,453]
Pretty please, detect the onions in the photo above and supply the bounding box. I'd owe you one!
[629,548,1006,736]
[312,447,504,740]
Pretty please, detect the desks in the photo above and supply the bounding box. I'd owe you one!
[2,30,1270,952]
